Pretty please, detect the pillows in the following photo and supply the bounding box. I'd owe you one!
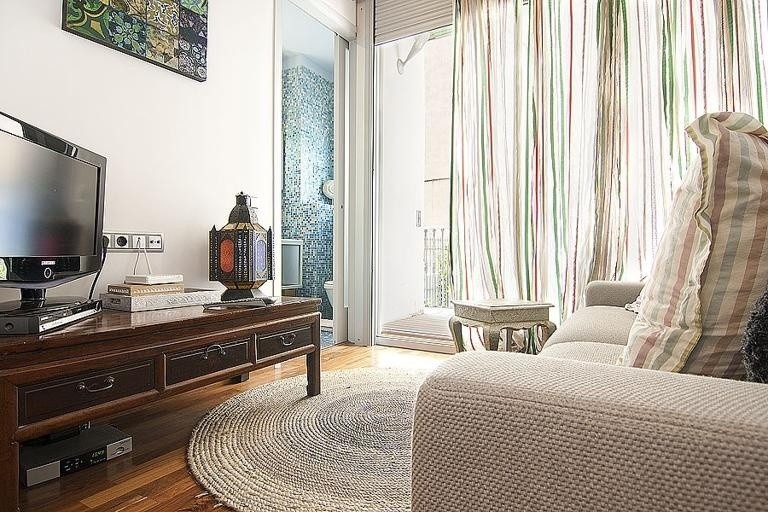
[618,109,768,381]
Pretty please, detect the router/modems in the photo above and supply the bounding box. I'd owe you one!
[125,248,184,285]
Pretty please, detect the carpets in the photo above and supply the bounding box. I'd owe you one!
[188,367,423,510]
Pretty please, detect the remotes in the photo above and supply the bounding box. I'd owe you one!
[203,297,267,309]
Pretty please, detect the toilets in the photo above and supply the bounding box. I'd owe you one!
[324,281,333,307]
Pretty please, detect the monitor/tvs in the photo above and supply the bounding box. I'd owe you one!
[0,111,108,307]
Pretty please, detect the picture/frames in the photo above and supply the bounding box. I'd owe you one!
[62,0,209,82]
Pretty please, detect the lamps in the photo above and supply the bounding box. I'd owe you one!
[209,190,275,300]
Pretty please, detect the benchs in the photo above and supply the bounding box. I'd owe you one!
[412,278,768,511]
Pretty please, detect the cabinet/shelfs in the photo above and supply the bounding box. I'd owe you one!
[0,293,323,494]
